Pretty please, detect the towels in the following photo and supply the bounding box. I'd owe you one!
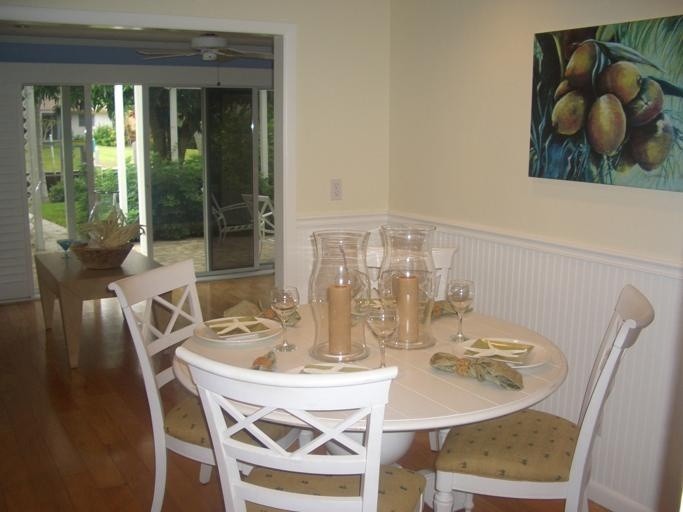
[246,347,278,374]
[424,348,525,393]
[219,296,302,328]
[417,294,475,325]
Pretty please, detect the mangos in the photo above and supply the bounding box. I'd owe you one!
[550,40,673,174]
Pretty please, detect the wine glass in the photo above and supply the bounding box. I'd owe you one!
[56,238,72,259]
[269,287,300,353]
[366,311,398,370]
[445,278,475,343]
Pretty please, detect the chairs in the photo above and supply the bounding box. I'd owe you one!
[107,256,300,512]
[327,243,456,450]
[175,347,426,512]
[200,186,254,253]
[431,285,653,512]
[242,193,276,264]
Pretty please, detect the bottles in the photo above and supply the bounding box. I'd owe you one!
[378,221,438,349]
[307,228,370,364]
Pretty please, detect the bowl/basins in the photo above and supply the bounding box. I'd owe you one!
[69,244,136,270]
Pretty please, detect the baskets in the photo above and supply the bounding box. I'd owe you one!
[70,241,134,269]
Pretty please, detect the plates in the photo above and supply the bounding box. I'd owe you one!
[286,363,375,375]
[461,337,546,370]
[194,317,283,344]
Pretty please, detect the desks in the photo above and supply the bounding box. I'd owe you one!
[168,311,568,510]
[35,249,175,370]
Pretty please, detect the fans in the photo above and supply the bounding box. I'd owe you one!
[133,30,273,65]
